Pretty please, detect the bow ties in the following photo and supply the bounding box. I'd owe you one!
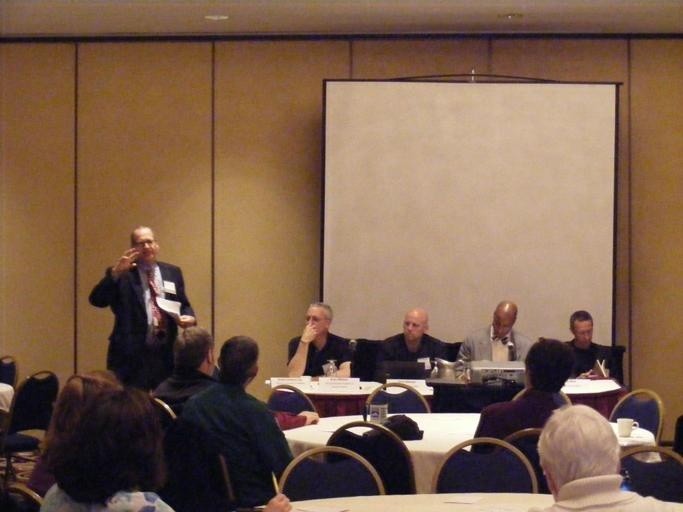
[492,335,510,345]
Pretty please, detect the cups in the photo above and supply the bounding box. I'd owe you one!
[617,418,639,437]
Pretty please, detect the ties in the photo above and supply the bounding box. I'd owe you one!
[147,270,166,340]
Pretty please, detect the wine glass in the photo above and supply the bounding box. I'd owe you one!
[430,361,440,378]
[327,360,337,377]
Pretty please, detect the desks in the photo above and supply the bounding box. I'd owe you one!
[283,412,655,493]
[267,377,629,417]
[289,493,554,511]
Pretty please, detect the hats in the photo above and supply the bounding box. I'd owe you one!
[363,415,423,440]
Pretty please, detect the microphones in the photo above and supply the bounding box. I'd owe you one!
[508,341,516,360]
[349,340,356,358]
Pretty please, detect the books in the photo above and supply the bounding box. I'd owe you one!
[591,359,607,380]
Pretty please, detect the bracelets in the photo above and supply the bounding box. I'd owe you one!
[300,340,307,344]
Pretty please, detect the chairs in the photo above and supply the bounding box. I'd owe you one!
[608,389,666,445]
[504,427,552,494]
[278,446,386,495]
[0,370,60,484]
[154,397,177,420]
[365,383,432,414]
[0,355,19,387]
[268,384,316,413]
[1,484,44,512]
[432,437,539,494]
[0,383,14,436]
[620,446,683,503]
[323,421,417,494]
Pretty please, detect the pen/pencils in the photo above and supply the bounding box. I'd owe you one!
[272,472,280,495]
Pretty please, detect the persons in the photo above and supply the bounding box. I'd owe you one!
[89,224,198,395]
[162,335,310,512]
[38,388,176,512]
[1,355,14,413]
[372,309,448,383]
[453,300,533,382]
[562,310,621,383]
[22,369,121,511]
[286,303,354,380]
[532,404,683,512]
[471,337,574,465]
[147,325,218,443]
